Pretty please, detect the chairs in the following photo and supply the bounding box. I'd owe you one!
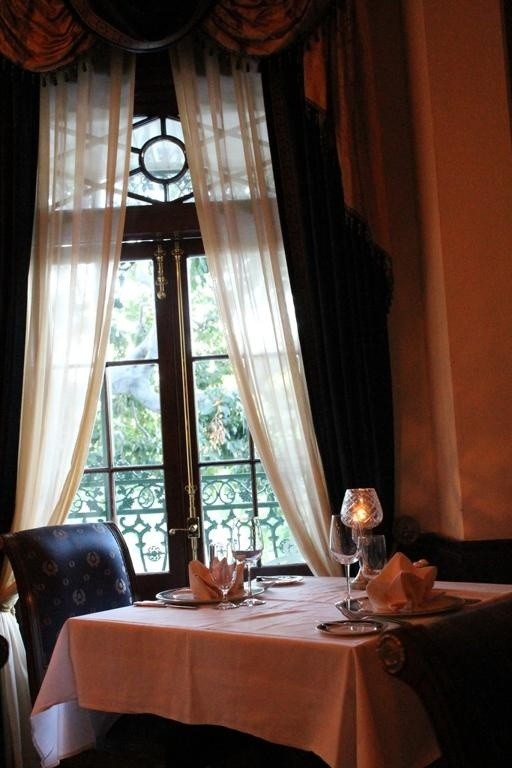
[2,523,166,767]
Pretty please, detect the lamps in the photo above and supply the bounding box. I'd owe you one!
[341,486,384,588]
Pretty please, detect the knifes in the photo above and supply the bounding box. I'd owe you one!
[134,600,198,610]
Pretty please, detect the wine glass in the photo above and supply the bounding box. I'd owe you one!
[324,515,389,613]
[209,518,264,610]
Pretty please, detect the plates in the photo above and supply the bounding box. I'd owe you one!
[156,576,304,604]
[317,594,465,636]
